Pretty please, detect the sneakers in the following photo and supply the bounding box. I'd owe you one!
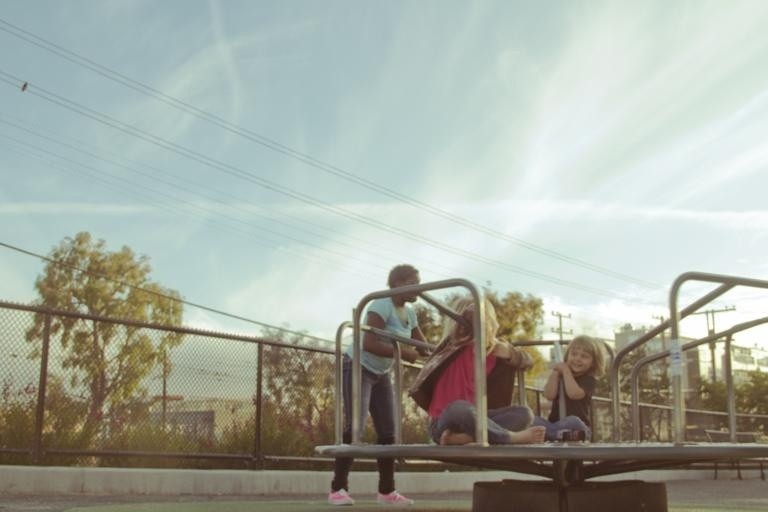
[327,488,354,505]
[563,430,586,441]
[376,491,414,505]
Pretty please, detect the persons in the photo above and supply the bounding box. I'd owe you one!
[405,294,547,448]
[525,333,606,444]
[326,263,431,506]
